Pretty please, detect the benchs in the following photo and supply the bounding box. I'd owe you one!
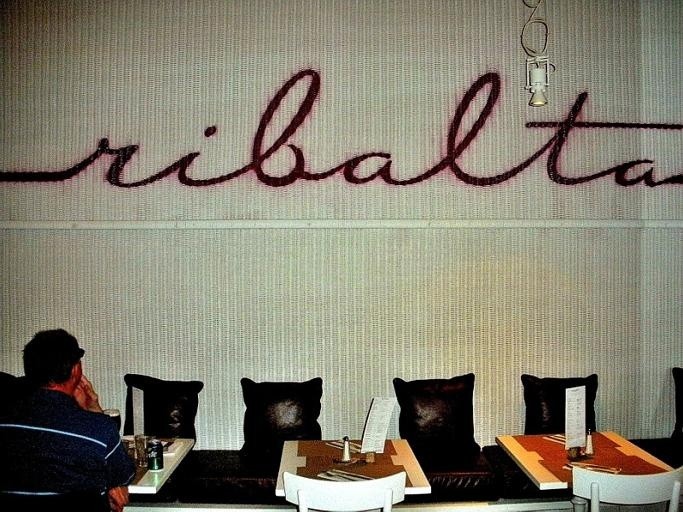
[482,436,681,511]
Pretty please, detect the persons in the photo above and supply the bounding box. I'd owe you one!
[0,329,136,512]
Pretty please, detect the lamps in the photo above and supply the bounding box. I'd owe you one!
[518,0,558,108]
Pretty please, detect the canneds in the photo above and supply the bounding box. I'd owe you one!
[147,439,163,470]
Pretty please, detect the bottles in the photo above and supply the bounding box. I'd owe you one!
[584,427,595,455]
[339,435,351,462]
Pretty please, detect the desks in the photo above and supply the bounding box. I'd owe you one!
[107,439,503,510]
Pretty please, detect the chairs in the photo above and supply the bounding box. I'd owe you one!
[281,470,408,512]
[517,371,599,432]
[234,374,322,451]
[392,373,479,447]
[114,374,203,441]
[668,366,682,442]
[568,462,683,510]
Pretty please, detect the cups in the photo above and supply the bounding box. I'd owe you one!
[102,408,120,433]
[120,432,155,470]
